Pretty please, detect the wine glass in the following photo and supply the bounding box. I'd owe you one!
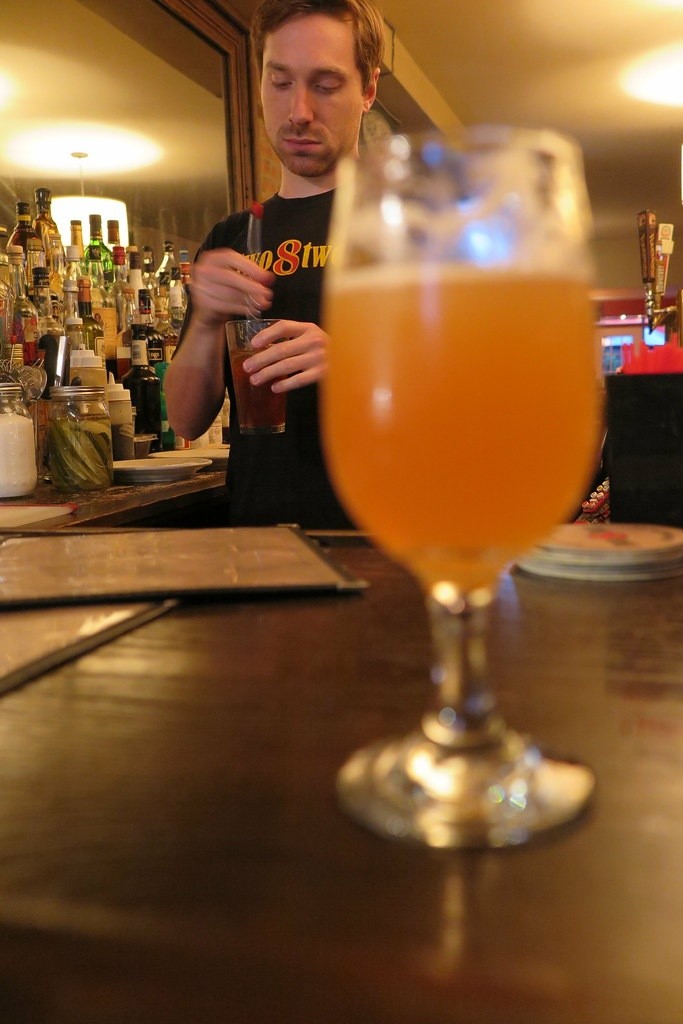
[319,123,602,848]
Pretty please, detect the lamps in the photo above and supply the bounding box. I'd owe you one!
[49,150,130,249]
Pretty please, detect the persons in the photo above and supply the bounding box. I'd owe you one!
[163,0,384,530]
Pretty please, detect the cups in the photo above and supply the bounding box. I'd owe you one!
[225,319,287,437]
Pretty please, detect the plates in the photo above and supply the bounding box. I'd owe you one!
[112,458,213,481]
[148,451,230,469]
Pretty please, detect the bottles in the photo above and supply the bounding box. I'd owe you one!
[0,187,106,370]
[106,373,135,460]
[121,331,162,454]
[84,214,191,383]
[46,385,112,493]
[174,388,230,450]
[154,361,175,450]
[1,245,40,383]
[0,383,37,498]
[63,318,107,386]
